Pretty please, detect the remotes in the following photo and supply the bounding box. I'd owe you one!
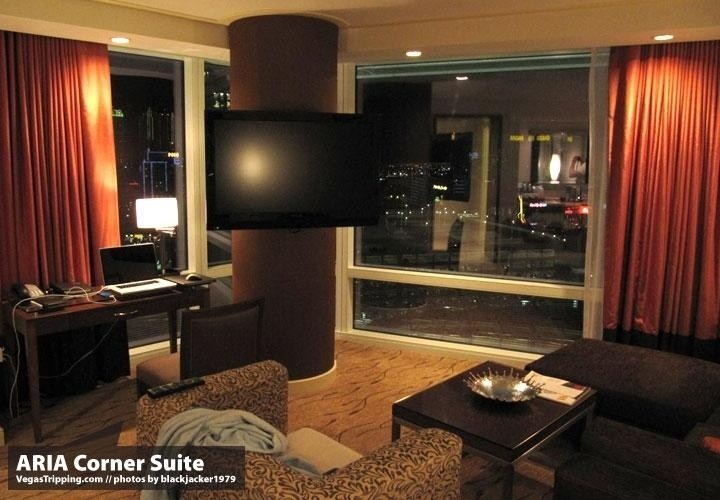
[146,376,205,399]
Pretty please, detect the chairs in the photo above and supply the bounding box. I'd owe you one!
[135,296,268,397]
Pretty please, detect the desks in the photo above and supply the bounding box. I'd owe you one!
[1,271,218,444]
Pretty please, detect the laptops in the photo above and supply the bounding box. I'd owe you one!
[98,242,177,300]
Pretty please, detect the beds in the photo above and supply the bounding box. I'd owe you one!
[392,361,600,500]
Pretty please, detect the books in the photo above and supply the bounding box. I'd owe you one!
[524,369,591,407]
[52,280,92,295]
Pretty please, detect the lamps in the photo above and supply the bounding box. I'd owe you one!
[549,155,561,182]
[135,197,180,275]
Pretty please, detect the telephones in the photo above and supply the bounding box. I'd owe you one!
[10,282,45,300]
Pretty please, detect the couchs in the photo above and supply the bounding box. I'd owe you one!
[134,359,465,500]
[522,337,719,500]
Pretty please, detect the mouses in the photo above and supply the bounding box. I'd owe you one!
[186,273,203,281]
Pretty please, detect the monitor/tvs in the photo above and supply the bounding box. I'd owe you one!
[204,111,384,231]
